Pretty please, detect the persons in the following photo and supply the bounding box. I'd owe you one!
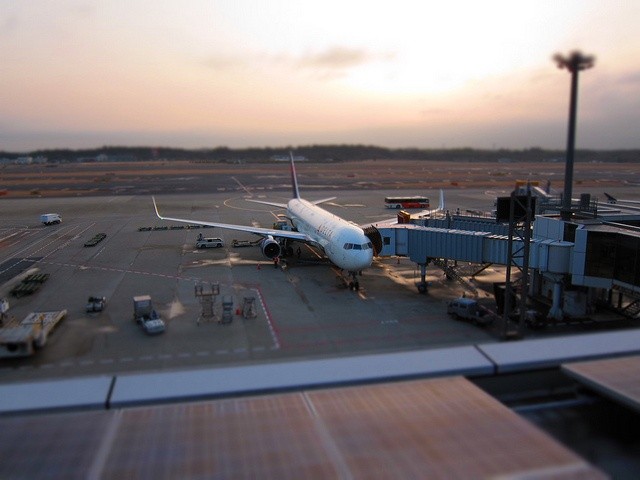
[256,263,261,272]
[456,208,460,216]
[296,246,302,258]
[198,231,205,240]
[273,256,279,268]
[445,210,450,217]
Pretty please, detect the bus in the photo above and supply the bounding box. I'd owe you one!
[384,196,430,208]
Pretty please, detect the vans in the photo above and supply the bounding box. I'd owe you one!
[41,213,62,225]
[196,238,224,248]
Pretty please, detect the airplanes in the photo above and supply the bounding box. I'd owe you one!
[152,151,445,292]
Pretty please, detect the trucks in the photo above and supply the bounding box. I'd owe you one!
[447,298,494,326]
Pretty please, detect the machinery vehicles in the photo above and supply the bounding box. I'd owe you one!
[133,295,165,334]
[85,295,106,314]
